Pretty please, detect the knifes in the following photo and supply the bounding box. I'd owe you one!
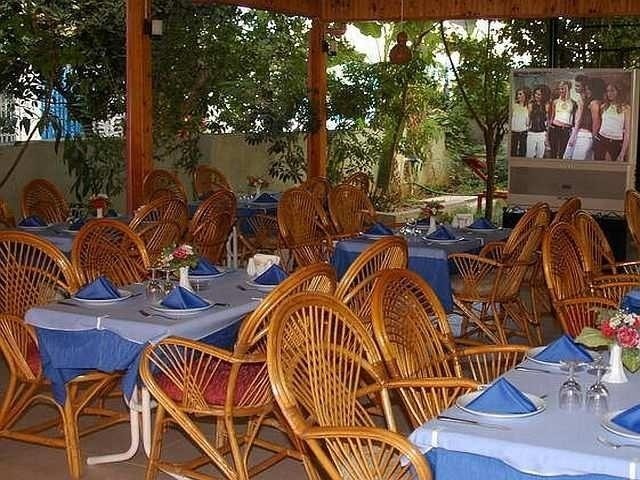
[514,366,577,377]
[437,416,512,432]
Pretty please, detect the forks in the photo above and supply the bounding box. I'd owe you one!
[596,438,640,449]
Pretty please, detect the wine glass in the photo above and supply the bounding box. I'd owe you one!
[558,358,611,413]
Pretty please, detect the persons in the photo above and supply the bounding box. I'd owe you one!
[510,72,634,162]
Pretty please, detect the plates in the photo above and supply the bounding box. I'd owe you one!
[359,224,503,243]
[57,264,280,321]
[16,223,54,231]
[457,392,547,419]
[526,346,601,368]
[63,230,79,235]
[600,410,640,440]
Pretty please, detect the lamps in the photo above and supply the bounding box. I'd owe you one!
[323,36,337,59]
[142,12,166,41]
